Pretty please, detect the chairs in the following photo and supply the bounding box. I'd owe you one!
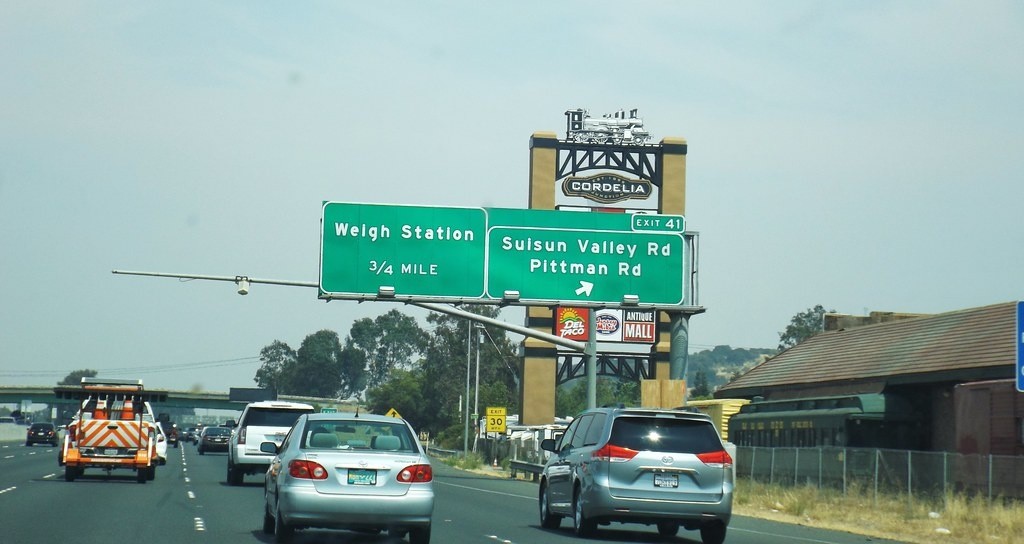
[312,432,339,448]
[374,435,401,450]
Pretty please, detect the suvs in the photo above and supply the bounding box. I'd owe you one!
[227,400,315,486]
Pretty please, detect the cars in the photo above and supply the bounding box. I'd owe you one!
[198,426,232,454]
[26,422,61,447]
[264,399,435,544]
[178,422,218,445]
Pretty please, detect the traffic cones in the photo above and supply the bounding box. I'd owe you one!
[121,400,134,420]
[94,402,107,419]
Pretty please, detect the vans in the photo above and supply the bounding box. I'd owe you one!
[538,406,733,544]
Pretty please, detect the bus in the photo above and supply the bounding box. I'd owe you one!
[728,393,922,491]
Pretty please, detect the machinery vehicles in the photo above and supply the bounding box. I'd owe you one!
[58,376,167,483]
[155,412,176,443]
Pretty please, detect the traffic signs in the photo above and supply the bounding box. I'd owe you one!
[318,200,690,310]
